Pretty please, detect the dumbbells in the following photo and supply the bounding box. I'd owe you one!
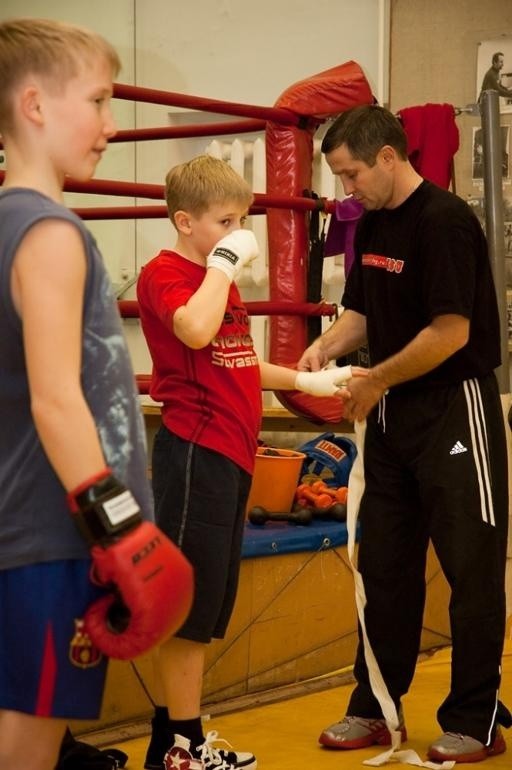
[248,506,311,526]
[292,480,348,522]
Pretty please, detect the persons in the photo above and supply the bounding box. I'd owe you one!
[479,52,512,101]
[0,15,197,769]
[296,103,510,761]
[138,151,371,770]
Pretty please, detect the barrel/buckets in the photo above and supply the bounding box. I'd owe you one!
[244,446,307,520]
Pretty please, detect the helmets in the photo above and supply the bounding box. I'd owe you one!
[297,433,357,487]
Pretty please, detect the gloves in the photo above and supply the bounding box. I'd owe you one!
[65,468,197,657]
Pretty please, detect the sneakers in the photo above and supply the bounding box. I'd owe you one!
[428,719,506,763]
[144,716,257,770]
[318,703,407,749]
